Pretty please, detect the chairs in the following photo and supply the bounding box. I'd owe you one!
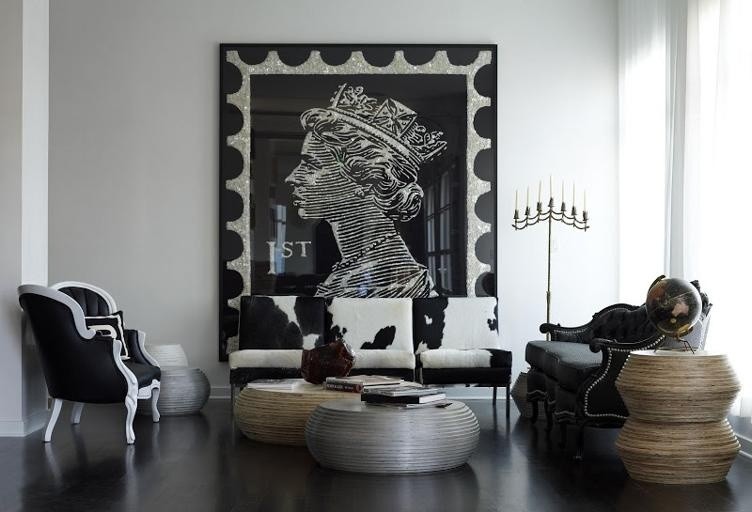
[523,276,710,457]
[15,274,163,443]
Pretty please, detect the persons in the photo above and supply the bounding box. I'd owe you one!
[284,84,448,299]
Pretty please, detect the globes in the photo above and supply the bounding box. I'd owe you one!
[645,274,703,354]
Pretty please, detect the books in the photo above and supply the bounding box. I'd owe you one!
[323,374,446,409]
[247,378,297,388]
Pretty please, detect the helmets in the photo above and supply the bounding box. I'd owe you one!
[301,339,356,385]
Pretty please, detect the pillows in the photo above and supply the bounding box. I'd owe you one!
[78,308,132,362]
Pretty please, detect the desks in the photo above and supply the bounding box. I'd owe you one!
[230,377,481,476]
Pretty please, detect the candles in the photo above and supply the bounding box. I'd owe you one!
[513,173,591,210]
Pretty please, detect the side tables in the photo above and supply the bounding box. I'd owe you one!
[615,350,744,486]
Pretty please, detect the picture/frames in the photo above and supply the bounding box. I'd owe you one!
[215,42,499,374]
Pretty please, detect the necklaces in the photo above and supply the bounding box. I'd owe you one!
[332,231,400,271]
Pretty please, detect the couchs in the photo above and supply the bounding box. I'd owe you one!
[227,295,513,425]
